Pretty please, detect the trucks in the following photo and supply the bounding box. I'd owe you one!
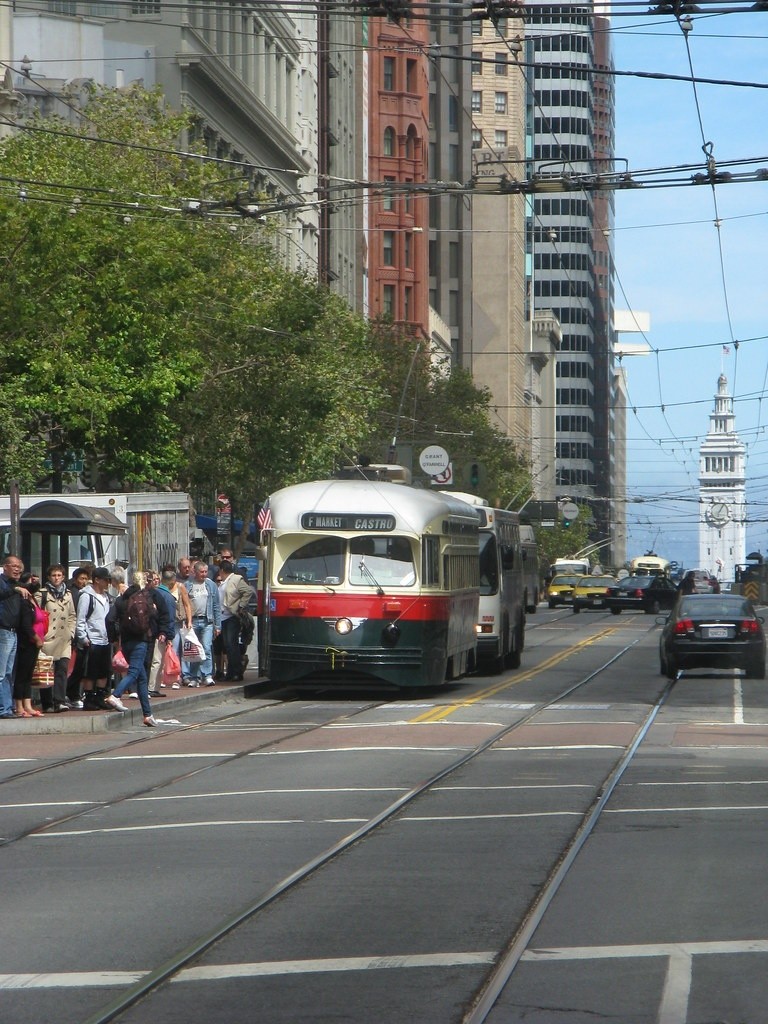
[0,492,190,583]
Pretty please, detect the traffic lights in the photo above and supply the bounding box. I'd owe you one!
[562,521,571,526]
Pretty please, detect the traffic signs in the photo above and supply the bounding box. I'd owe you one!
[43,460,84,472]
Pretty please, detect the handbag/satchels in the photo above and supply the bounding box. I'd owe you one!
[111,651,129,673]
[163,644,182,676]
[31,651,54,689]
[180,628,206,662]
[41,610,50,636]
[176,597,197,621]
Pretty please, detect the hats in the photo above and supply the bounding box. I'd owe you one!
[92,567,113,580]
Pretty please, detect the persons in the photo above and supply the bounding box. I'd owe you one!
[677,570,697,599]
[352,539,393,578]
[0,546,255,726]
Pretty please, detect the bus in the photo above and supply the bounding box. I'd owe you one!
[257,464,480,696]
[441,489,525,670]
[519,526,540,613]
[549,558,591,576]
[629,556,671,580]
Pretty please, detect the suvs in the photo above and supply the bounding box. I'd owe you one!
[683,569,713,594]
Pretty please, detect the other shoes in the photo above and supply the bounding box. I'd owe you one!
[0,713,17,719]
[129,692,138,699]
[160,683,167,688]
[150,691,166,697]
[143,714,158,727]
[30,711,44,717]
[105,695,129,712]
[53,703,70,713]
[213,670,247,685]
[71,701,84,708]
[172,682,181,690]
[42,707,55,713]
[15,711,33,718]
[203,678,215,686]
[187,680,197,688]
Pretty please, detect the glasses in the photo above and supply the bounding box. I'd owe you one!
[221,556,230,559]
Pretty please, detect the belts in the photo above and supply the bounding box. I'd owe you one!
[193,616,207,619]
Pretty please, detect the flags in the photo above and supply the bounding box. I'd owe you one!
[257,496,273,536]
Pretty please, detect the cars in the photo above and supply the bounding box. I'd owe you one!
[605,576,679,614]
[546,564,584,608]
[570,565,618,613]
[655,594,766,679]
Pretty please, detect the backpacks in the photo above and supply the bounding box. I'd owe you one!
[121,586,154,640]
